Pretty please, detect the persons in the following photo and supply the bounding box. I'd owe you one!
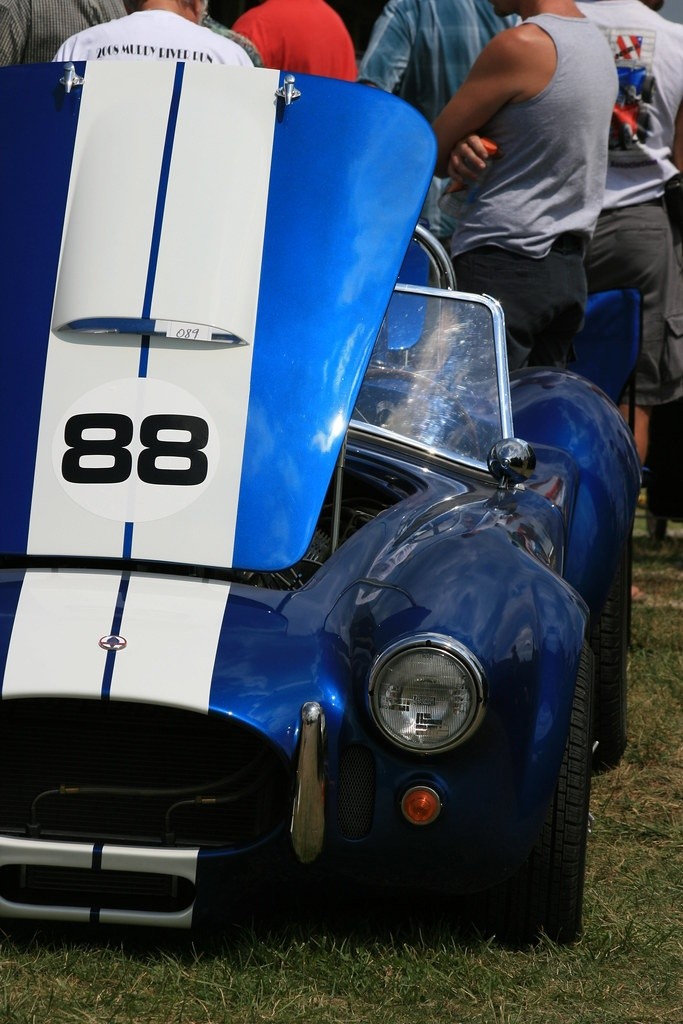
[0,0,682,609]
[431,0,619,376]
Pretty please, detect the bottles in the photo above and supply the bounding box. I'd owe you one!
[438,136,497,221]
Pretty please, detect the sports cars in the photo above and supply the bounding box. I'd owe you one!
[0,53,646,960]
